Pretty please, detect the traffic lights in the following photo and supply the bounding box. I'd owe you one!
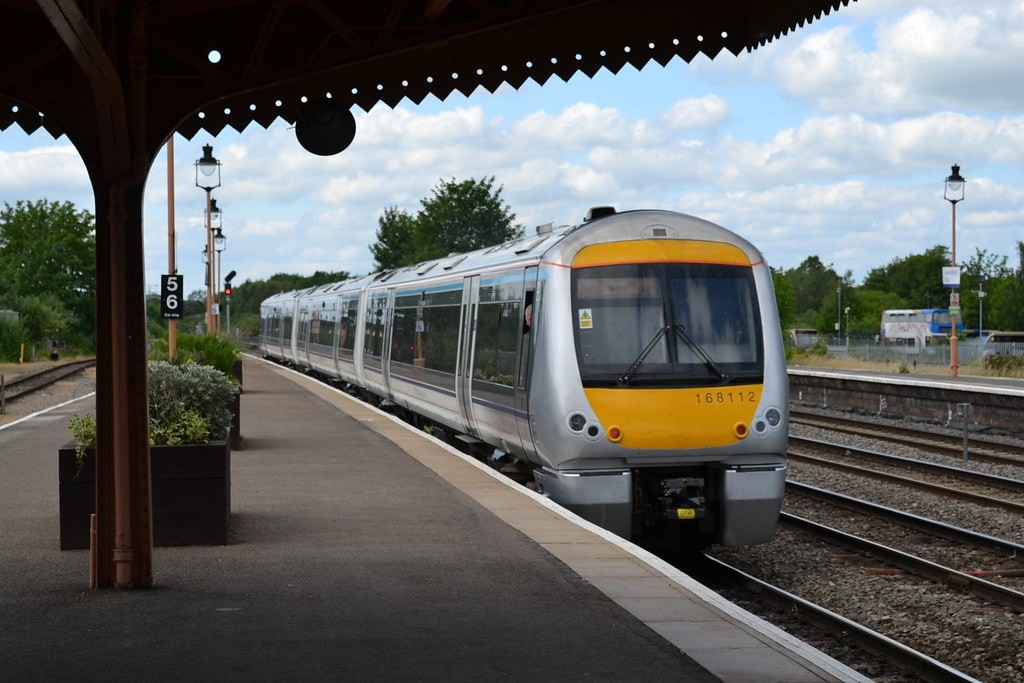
[225,283,232,296]
[203,196,223,335]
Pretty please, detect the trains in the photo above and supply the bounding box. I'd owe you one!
[257,207,790,559]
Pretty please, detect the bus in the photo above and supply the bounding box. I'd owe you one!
[880,308,964,344]
[980,330,1023,343]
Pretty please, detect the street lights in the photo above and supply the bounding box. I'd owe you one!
[975,276,986,340]
[844,305,851,347]
[212,228,227,334]
[944,164,966,378]
[195,143,222,336]
[202,242,216,335]
